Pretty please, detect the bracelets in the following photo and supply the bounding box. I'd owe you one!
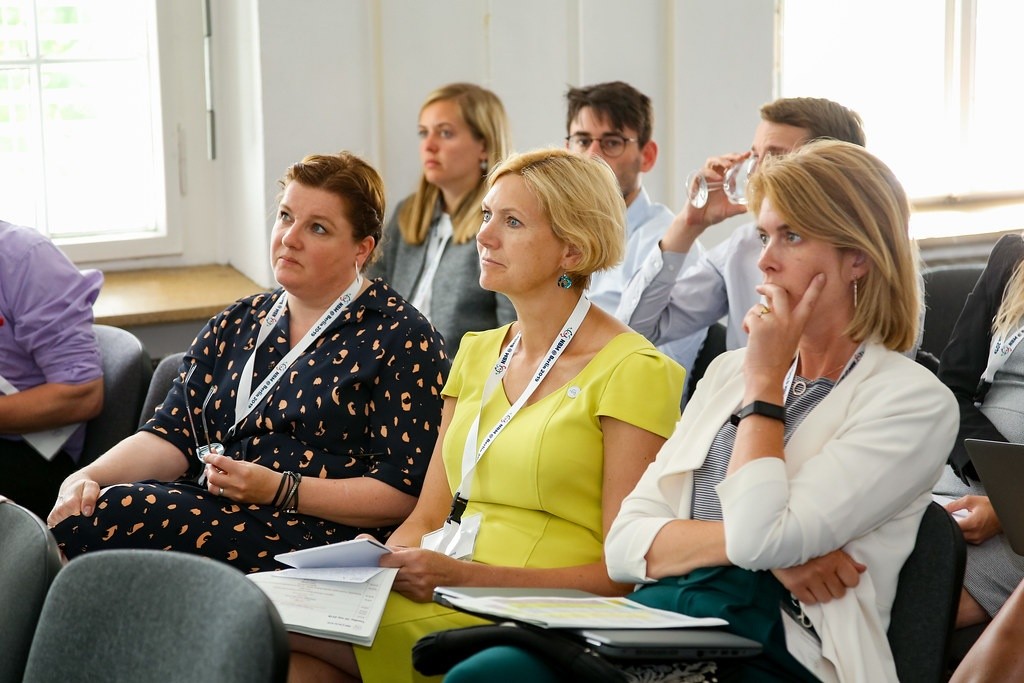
[731,400,787,426]
[270,470,302,513]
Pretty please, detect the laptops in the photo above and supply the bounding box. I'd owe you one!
[963,438,1024,555]
[432,586,766,657]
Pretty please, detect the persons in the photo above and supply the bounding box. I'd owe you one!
[44,151,451,580]
[363,81,517,358]
[563,81,927,415]
[287,149,686,683]
[0,219,104,515]
[440,138,961,683]
[934,229,1024,683]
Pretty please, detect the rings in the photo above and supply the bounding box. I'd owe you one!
[219,488,223,496]
[761,307,771,317]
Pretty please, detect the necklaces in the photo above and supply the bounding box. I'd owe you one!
[793,354,845,396]
[413,206,455,320]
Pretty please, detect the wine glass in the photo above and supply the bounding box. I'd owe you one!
[686,156,757,208]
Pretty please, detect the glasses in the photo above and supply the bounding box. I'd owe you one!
[184,362,224,466]
[565,131,641,160]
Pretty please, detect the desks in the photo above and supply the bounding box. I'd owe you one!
[90,263,272,327]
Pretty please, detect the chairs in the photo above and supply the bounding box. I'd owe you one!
[0,267,999,683]
